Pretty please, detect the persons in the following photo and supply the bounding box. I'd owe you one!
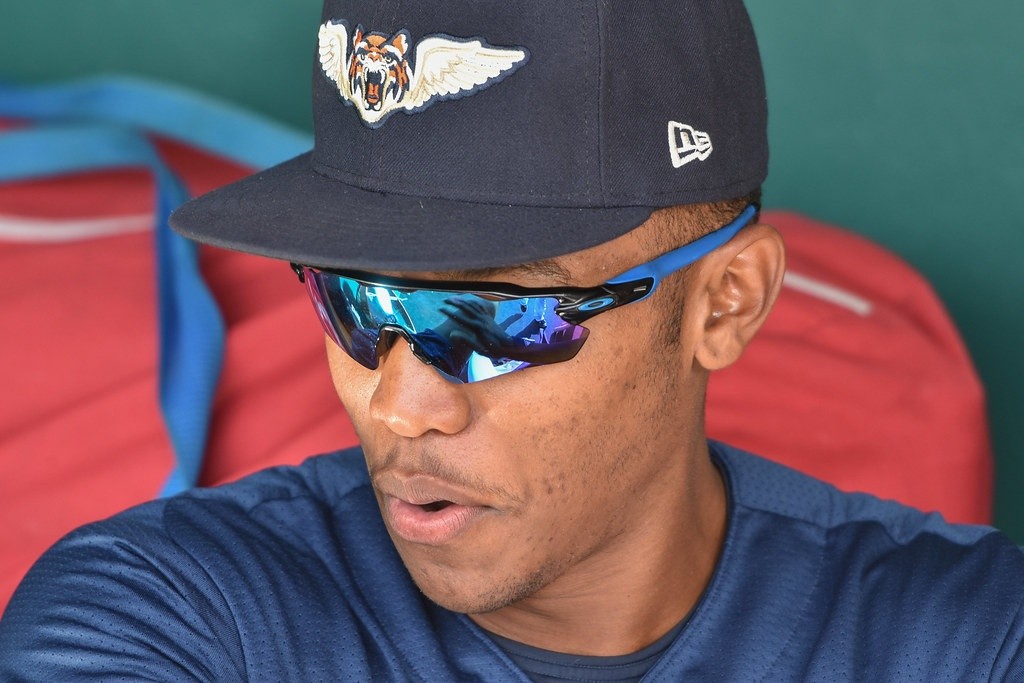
[1,1,1024,683]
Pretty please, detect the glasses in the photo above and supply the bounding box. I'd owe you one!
[288,204,758,384]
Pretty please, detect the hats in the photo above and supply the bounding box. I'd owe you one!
[168,0,768,272]
